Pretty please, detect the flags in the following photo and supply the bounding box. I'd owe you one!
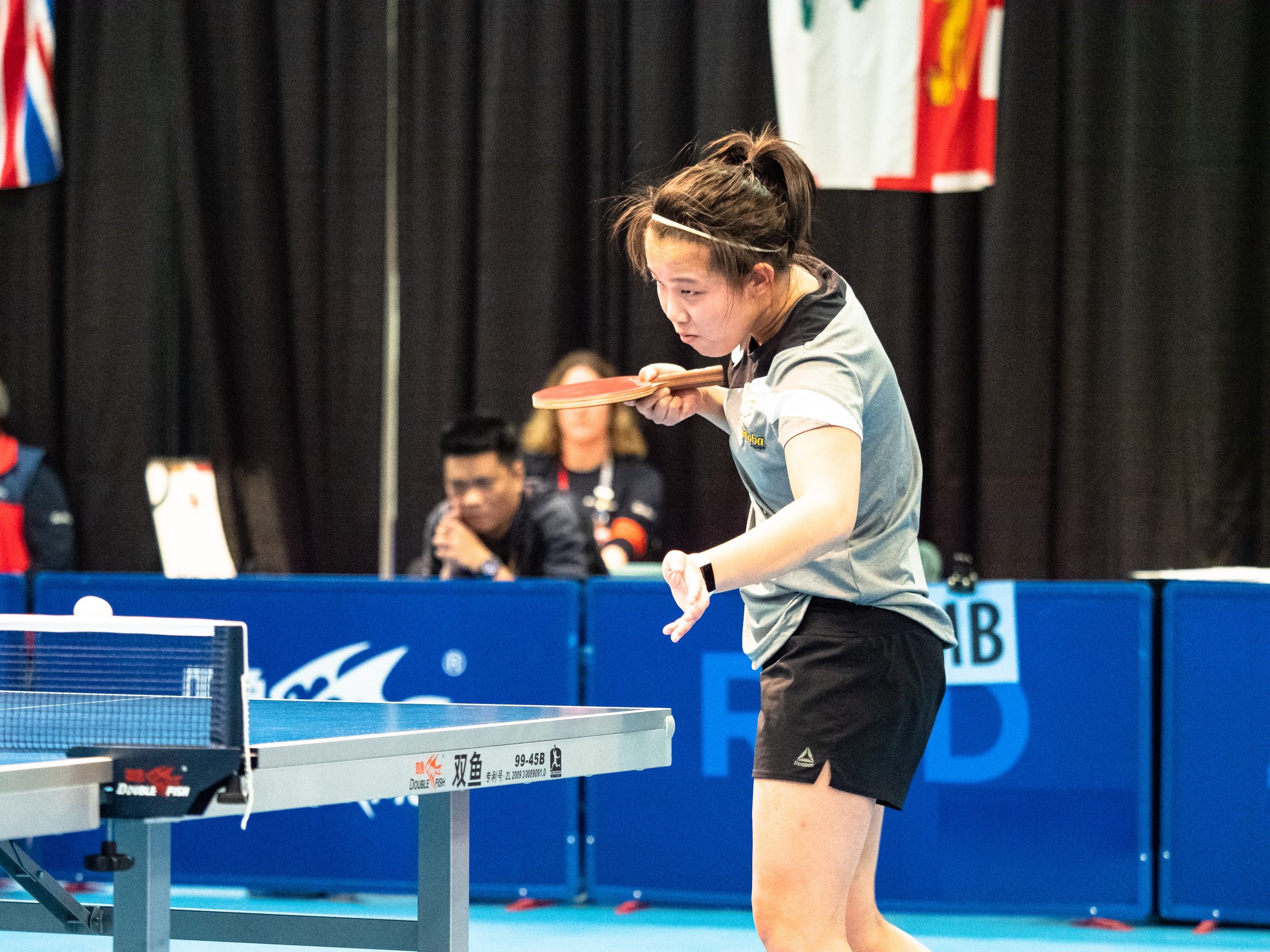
[766,0,1004,192]
[0,0,64,188]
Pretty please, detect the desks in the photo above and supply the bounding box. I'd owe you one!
[0,617,674,952]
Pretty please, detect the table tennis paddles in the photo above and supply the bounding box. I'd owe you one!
[532,364,725,412]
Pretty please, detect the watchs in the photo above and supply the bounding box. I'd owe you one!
[690,553,716,597]
[480,555,501,579]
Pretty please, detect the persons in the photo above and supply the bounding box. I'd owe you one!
[606,132,960,951]
[521,351,665,572]
[420,415,612,582]
[0,383,77,574]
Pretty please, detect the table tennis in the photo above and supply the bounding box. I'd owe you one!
[72,595,114,617]
